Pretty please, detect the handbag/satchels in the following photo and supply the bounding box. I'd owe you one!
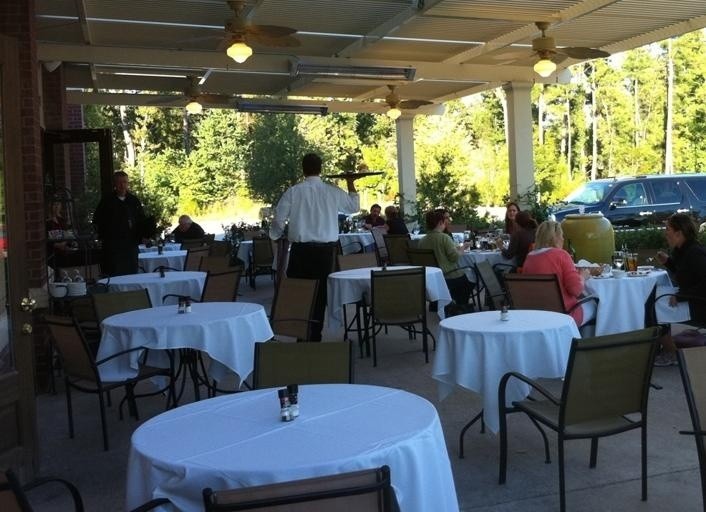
[672,329,706,348]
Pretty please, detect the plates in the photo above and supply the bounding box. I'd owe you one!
[48,282,86,297]
[628,271,651,277]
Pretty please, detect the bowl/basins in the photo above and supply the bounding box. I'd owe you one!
[611,270,625,279]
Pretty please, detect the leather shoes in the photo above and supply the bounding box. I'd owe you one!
[654,355,679,367]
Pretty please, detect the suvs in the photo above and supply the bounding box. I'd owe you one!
[546,173,705,232]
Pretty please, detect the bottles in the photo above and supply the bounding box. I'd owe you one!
[287,386,299,418]
[500,302,509,322]
[276,389,292,423]
[178,295,191,314]
[157,244,162,256]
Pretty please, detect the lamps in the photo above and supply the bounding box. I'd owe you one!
[182,102,203,115]
[224,42,254,65]
[531,58,557,77]
[386,108,402,121]
[288,59,416,82]
[237,101,329,117]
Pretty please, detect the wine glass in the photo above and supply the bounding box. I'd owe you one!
[169,233,175,246]
[461,230,510,251]
[613,251,625,270]
[346,219,365,233]
[412,223,419,240]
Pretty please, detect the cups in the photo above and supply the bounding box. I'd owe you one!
[627,254,638,271]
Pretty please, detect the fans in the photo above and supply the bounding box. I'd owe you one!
[147,75,228,106]
[381,84,433,109]
[173,0,297,43]
[518,22,611,64]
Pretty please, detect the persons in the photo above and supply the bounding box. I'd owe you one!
[382,205,409,236]
[521,221,598,329]
[418,212,477,318]
[359,204,385,234]
[169,216,206,242]
[496,209,540,276]
[427,208,454,243]
[653,212,705,369]
[502,202,519,237]
[91,171,148,275]
[269,154,360,343]
